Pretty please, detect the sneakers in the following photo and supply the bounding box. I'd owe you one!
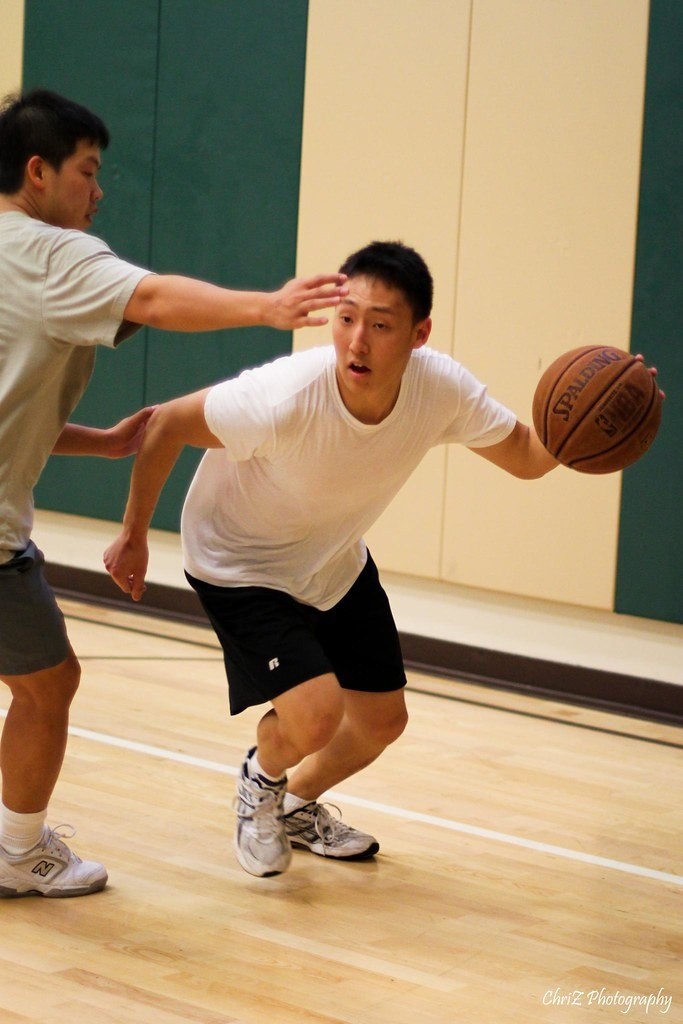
[0,824,108,899]
[232,746,292,878]
[284,801,380,861]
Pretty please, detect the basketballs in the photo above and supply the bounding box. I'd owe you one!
[532,346,665,477]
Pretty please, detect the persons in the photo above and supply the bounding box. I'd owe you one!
[103,242,665,879]
[0,90,349,898]
